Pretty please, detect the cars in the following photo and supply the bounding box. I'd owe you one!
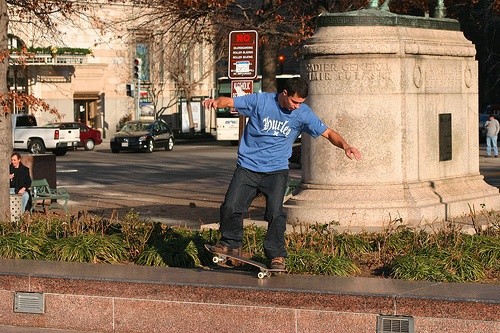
[46,122,102,151]
[110,120,175,153]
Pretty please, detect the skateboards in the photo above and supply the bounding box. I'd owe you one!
[203,243,287,278]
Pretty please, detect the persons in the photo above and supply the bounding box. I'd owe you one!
[9,153,33,214]
[202,78,362,270]
[484,114,500,158]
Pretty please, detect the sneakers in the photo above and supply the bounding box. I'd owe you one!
[211,243,240,254]
[271,257,286,269]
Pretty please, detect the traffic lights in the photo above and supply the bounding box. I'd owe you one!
[126,84,135,98]
[134,59,142,79]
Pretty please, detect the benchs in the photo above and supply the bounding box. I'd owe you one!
[28,180,70,214]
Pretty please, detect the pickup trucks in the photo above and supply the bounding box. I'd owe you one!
[11,113,81,156]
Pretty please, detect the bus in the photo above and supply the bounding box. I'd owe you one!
[213,74,302,145]
